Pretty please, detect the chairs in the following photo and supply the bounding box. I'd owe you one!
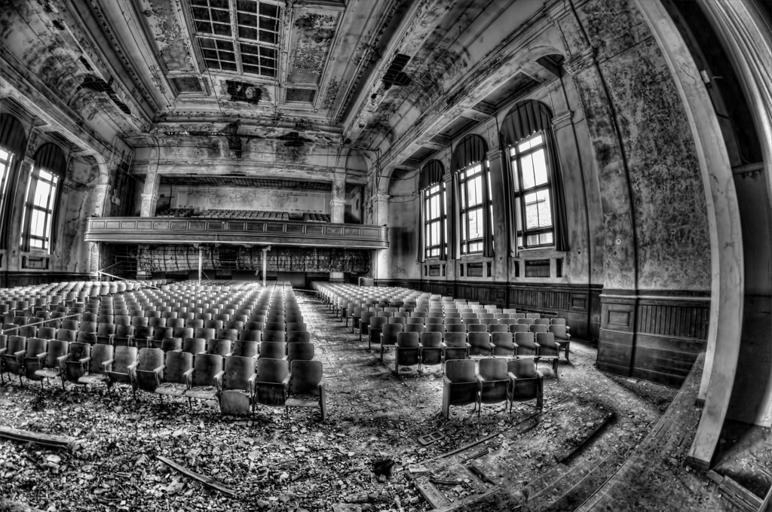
[332,289,574,425]
[2,280,329,425]
